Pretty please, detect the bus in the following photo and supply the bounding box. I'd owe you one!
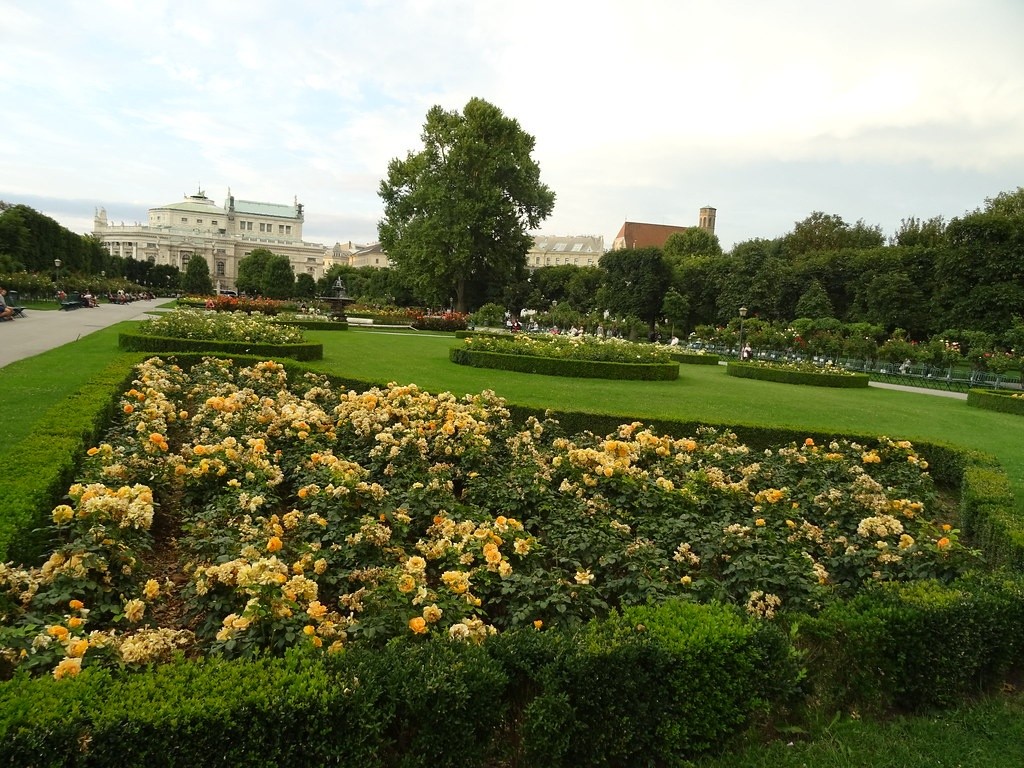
[213,289,237,297]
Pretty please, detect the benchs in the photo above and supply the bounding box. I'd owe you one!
[688,342,1024,394]
[0,293,142,320]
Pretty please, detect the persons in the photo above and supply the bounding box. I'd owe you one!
[0,289,15,321]
[668,335,680,346]
[741,341,752,360]
[83,289,156,308]
[506,317,540,333]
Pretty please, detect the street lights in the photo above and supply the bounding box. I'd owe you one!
[101,270,106,298]
[54,259,62,301]
[124,276,127,294]
[738,306,748,359]
